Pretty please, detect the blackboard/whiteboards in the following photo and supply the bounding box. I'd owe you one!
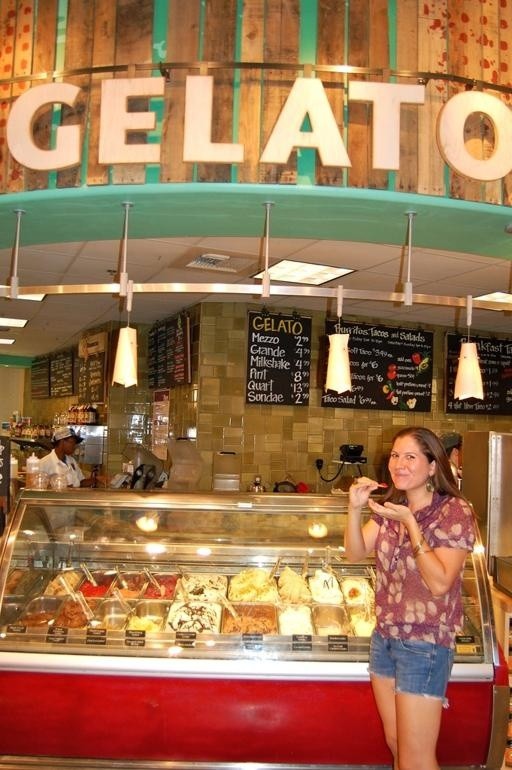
[30,346,107,405]
[320,318,434,412]
[147,311,191,389]
[246,310,312,406]
[444,332,512,416]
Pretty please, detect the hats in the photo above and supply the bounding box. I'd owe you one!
[439,431,463,448]
[51,427,84,444]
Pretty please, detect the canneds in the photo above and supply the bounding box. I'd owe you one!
[15,423,54,438]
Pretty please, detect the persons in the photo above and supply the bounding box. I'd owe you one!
[38,427,117,489]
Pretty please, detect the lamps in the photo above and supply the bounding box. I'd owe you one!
[259,199,274,298]
[326,288,353,396]
[454,293,487,402]
[400,211,415,306]
[109,275,142,389]
[11,207,25,299]
[119,200,135,295]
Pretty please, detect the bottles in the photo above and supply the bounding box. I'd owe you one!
[26,452,40,488]
[10,422,54,441]
[53,403,99,426]
[10,457,19,479]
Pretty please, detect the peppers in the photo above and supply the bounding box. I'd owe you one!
[385,388,395,399]
[412,352,421,365]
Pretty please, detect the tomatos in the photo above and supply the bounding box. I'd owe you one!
[387,363,398,379]
